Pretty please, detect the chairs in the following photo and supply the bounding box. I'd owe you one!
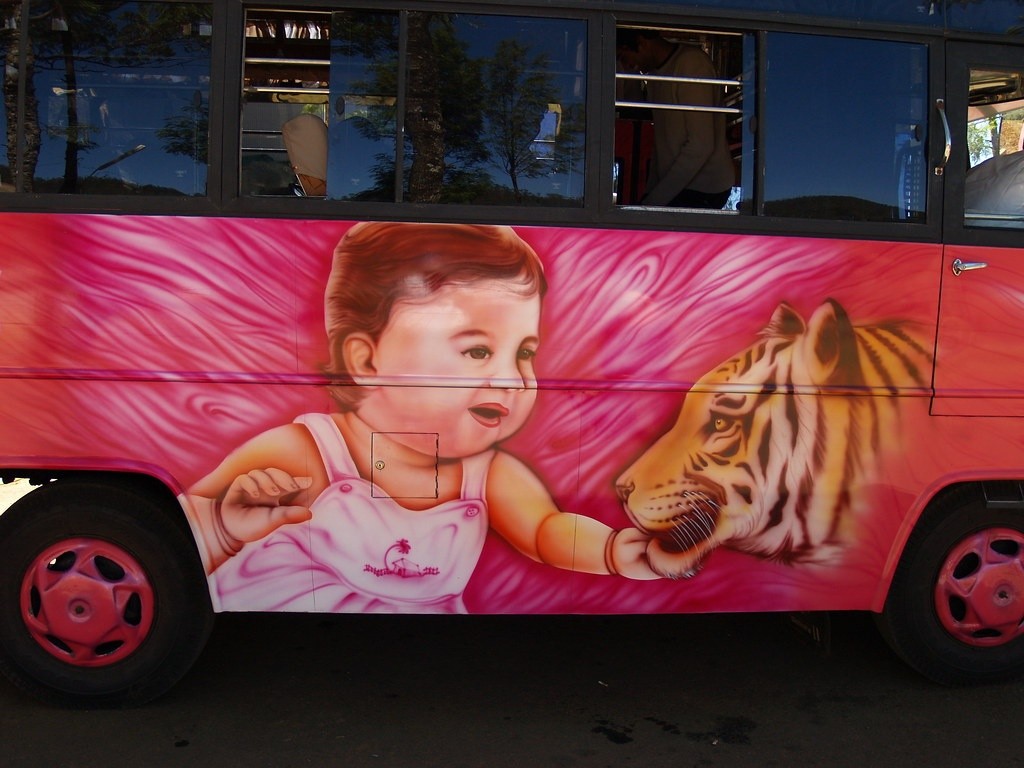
[282,114,328,199]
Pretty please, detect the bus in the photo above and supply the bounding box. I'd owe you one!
[1,1,1024,707]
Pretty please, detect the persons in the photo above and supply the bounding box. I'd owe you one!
[613,28,736,208]
